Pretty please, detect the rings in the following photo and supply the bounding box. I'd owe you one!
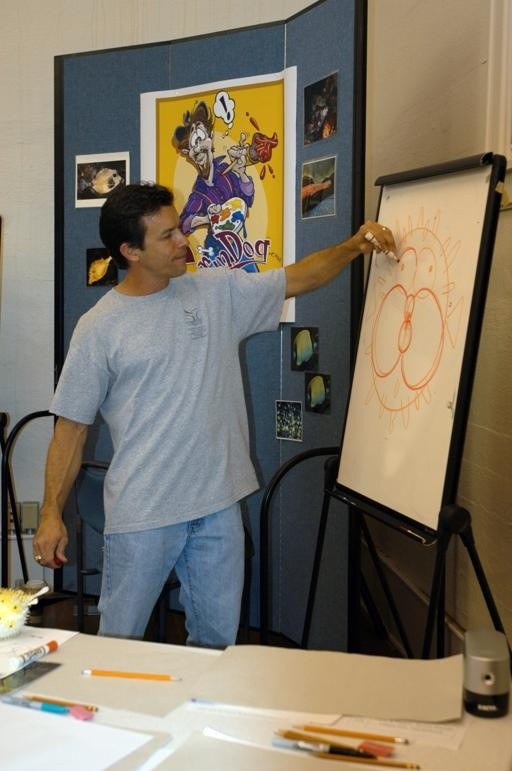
[7,641,59,668]
[25,696,100,715]
[273,725,425,769]
[84,669,184,685]
[365,233,400,263]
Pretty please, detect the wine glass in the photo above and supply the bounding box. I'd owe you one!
[381,227,387,231]
[34,554,42,562]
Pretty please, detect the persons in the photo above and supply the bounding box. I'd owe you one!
[32,183,393,649]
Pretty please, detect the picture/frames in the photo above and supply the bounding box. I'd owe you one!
[0,626,512,770]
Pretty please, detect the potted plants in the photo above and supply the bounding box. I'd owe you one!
[326,153,508,545]
